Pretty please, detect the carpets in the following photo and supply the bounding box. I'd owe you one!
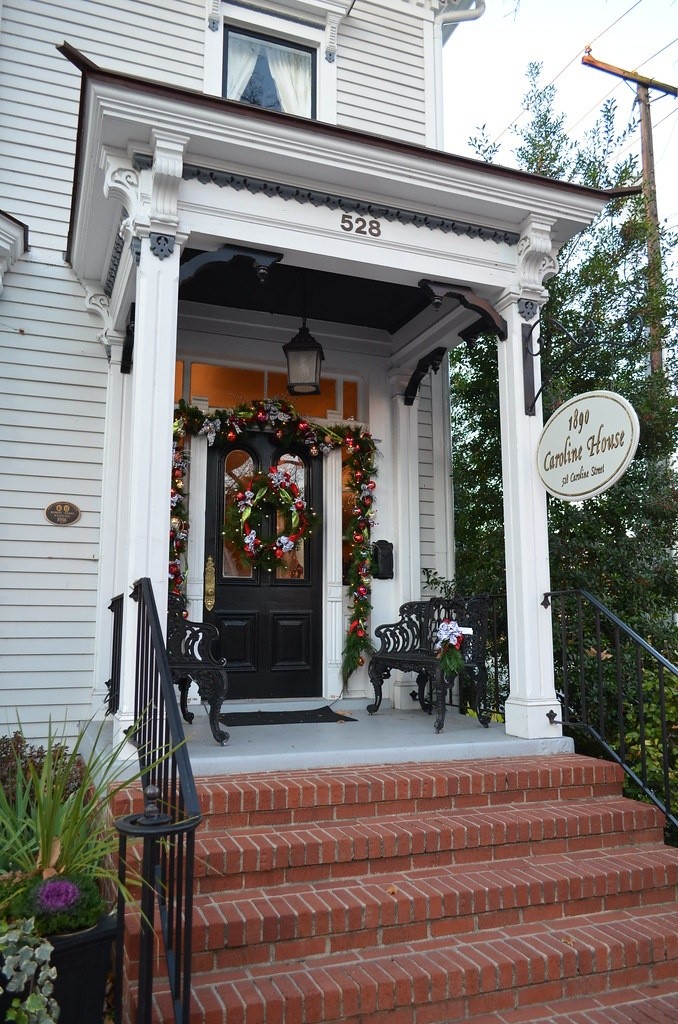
[218,706,358,727]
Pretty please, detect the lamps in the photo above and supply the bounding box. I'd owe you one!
[281,315,327,397]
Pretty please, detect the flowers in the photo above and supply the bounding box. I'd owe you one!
[0,694,221,972]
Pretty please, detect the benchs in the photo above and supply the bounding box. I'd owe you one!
[366,596,494,734]
[166,591,231,747]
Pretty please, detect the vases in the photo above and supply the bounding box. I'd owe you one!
[0,913,114,1024]
